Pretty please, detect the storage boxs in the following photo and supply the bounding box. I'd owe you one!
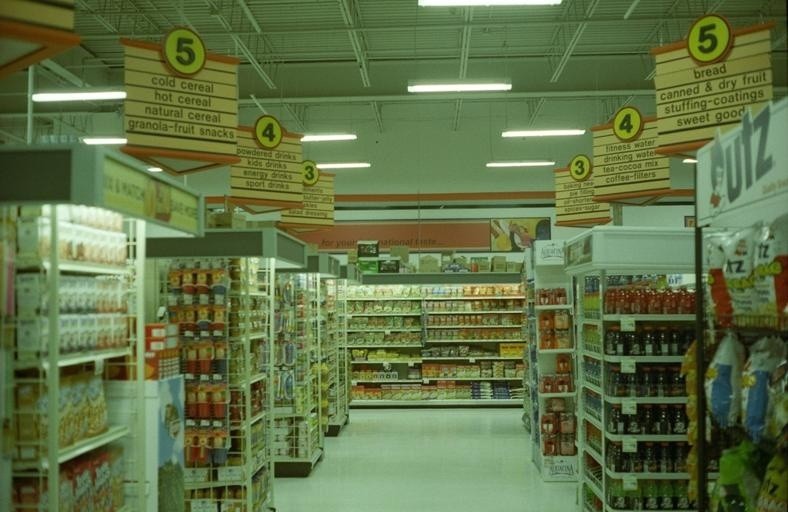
[350,240,521,274]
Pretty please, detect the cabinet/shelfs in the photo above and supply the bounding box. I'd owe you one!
[521,144,704,510]
[348,274,525,409]
[1,142,349,510]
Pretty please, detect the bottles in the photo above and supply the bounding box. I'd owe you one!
[604,324,696,511]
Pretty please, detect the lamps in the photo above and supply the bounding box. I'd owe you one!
[32,87,129,103]
[77,136,128,146]
[408,78,513,93]
[416,0,562,6]
[501,129,586,136]
[300,134,358,141]
